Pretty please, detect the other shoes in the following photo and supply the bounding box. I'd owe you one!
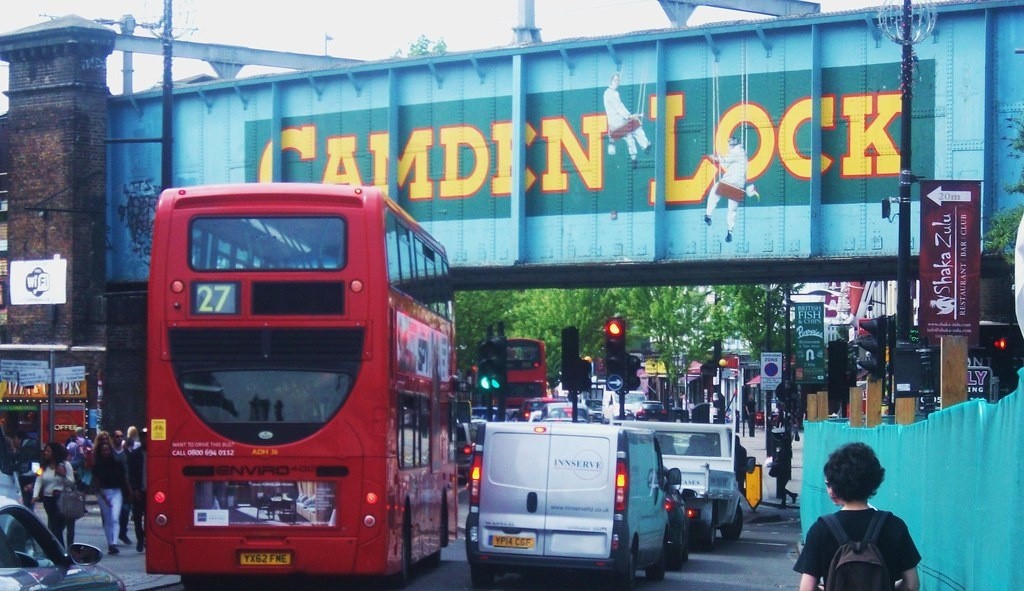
[791,492,798,504]
[777,504,787,509]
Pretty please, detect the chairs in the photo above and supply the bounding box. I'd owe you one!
[657,435,714,456]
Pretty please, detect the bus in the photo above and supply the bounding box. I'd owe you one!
[146,184,463,591]
[492,338,547,408]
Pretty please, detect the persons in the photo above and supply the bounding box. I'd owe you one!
[769,435,798,509]
[746,394,756,415]
[30,443,75,543]
[0,426,144,552]
[793,442,922,591]
[92,441,125,554]
[680,392,687,410]
[776,409,800,441]
[703,435,747,491]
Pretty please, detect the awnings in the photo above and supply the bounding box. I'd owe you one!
[678,377,699,384]
[746,375,760,384]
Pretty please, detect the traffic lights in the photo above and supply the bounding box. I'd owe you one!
[477,340,489,393]
[489,335,505,394]
[606,317,626,391]
[857,314,887,378]
[624,353,640,391]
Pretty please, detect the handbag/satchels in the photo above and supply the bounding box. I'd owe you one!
[52,488,86,520]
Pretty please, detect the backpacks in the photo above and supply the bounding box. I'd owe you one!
[818,509,894,591]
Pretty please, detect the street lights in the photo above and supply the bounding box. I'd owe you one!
[718,356,742,422]
[881,0,916,425]
[584,356,594,376]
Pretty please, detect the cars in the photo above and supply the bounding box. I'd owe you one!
[456,397,591,474]
[662,465,698,569]
[0,495,126,591]
[634,401,668,422]
[585,399,602,422]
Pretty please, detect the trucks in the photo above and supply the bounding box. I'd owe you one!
[466,422,681,591]
[622,420,756,551]
[602,391,647,425]
